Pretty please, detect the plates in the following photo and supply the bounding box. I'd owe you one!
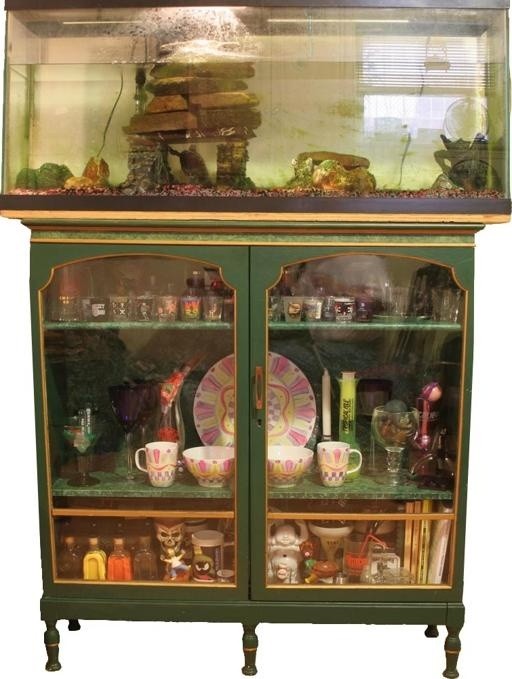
[193,349,317,447]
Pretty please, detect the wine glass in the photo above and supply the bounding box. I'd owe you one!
[370,406,420,485]
[62,380,159,487]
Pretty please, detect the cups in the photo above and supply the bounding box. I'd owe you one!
[343,534,372,583]
[192,530,235,582]
[54,294,225,323]
[134,442,178,487]
[317,440,363,487]
[268,286,466,325]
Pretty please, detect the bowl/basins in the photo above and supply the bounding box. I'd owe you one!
[267,446,314,487]
[183,446,235,488]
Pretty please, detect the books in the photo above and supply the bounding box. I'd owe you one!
[397,501,454,585]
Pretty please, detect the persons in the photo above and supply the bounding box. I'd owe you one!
[268,518,309,585]
[160,549,191,580]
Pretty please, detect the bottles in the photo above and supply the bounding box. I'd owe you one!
[57,535,158,581]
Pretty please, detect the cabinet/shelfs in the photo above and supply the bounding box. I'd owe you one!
[25,224,483,679]
